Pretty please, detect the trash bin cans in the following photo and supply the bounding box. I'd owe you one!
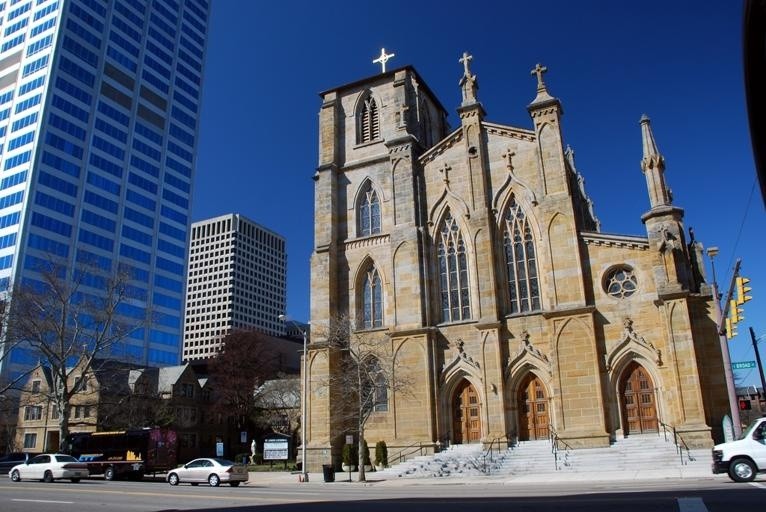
[323,464,335,482]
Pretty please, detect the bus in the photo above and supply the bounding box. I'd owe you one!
[61,426,177,481]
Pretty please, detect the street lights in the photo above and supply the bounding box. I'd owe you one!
[279,315,306,473]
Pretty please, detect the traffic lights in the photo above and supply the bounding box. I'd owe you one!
[735,277,752,306]
[725,318,738,339]
[739,399,752,411]
[730,300,745,323]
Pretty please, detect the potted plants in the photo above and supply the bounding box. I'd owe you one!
[342,439,387,472]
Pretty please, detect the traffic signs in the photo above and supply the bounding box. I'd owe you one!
[731,360,756,370]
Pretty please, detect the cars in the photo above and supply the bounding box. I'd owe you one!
[164,457,249,486]
[1,452,89,483]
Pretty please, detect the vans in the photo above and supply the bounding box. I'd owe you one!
[712,418,766,482]
